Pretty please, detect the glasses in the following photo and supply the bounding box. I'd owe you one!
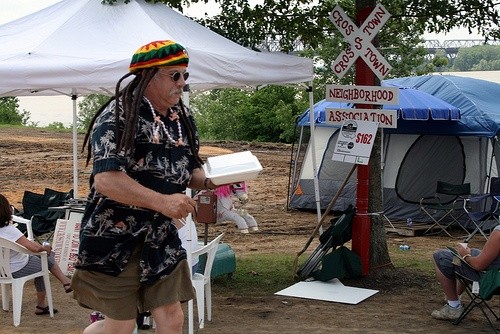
[157,71,189,82]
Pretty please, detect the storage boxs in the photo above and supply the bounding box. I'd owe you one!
[202,150,264,187]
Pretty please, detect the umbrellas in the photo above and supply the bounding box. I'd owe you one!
[298,82,461,198]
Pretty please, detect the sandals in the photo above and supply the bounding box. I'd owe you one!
[63,283,73,294]
[34,305,58,315]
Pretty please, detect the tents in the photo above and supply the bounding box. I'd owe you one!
[0,0,323,237]
[289,75,500,230]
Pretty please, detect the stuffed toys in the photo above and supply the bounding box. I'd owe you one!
[193,181,258,234]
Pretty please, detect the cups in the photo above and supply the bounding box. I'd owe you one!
[459,243,467,248]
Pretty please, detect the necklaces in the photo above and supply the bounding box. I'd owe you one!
[143,96,184,146]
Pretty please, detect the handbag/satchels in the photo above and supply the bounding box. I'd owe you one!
[311,244,362,281]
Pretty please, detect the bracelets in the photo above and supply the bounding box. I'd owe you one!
[204,178,208,187]
[463,254,471,259]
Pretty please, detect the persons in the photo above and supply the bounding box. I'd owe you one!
[431,225,500,320]
[73,41,228,334]
[0,194,73,316]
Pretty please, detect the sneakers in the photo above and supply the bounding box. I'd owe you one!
[444,294,462,305]
[431,303,465,320]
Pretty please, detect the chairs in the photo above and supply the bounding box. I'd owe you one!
[419,177,500,243]
[296,204,356,279]
[146,232,224,334]
[446,246,500,332]
[0,214,54,326]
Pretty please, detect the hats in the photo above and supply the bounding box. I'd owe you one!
[129,39,189,75]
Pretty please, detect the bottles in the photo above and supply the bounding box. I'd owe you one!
[43,239,50,246]
[399,244,410,251]
